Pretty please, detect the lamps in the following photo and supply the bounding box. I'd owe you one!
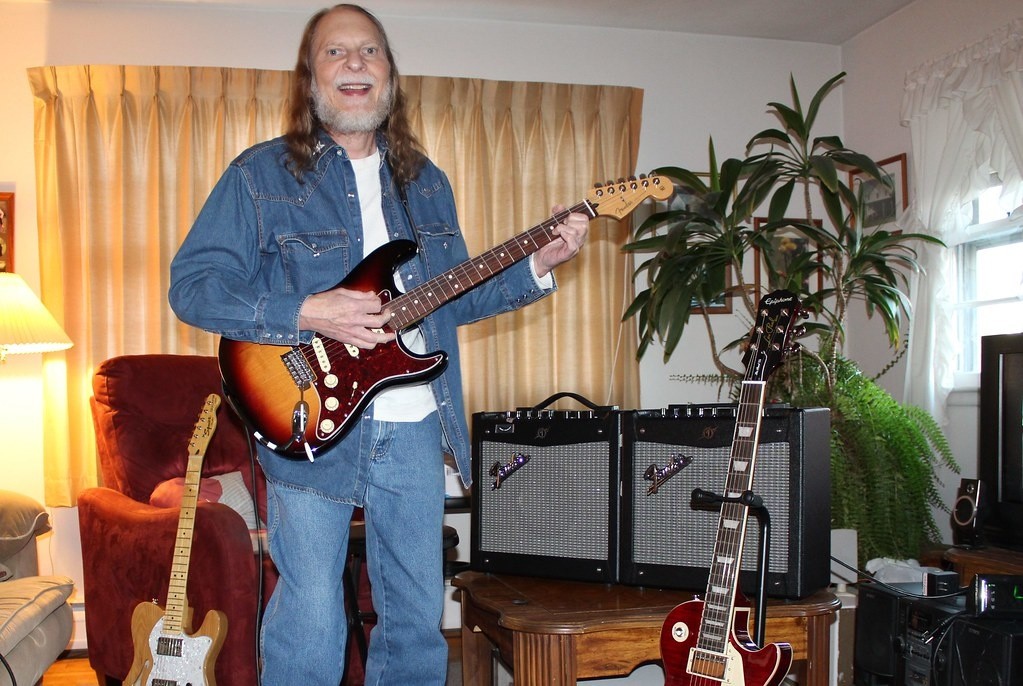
[0,272,74,365]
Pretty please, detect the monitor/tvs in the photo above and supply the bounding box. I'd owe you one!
[977,332,1023,529]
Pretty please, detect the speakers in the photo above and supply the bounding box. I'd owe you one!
[948,478,983,548]
[470,405,833,594]
[856,578,1023,686]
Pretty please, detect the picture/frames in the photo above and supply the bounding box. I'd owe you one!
[754,215,823,312]
[848,153,908,244]
[652,171,734,315]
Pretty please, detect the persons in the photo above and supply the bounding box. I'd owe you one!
[168,3,588,686]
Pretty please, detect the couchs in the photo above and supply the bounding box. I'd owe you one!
[0,486,76,686]
[78,353,460,686]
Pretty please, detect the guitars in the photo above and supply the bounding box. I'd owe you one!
[658,288,810,685]
[216,168,676,462]
[121,394,229,686]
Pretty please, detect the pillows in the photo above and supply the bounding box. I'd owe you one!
[148,470,270,554]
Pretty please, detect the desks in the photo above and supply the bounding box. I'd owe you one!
[943,545,1023,589]
[450,568,844,686]
